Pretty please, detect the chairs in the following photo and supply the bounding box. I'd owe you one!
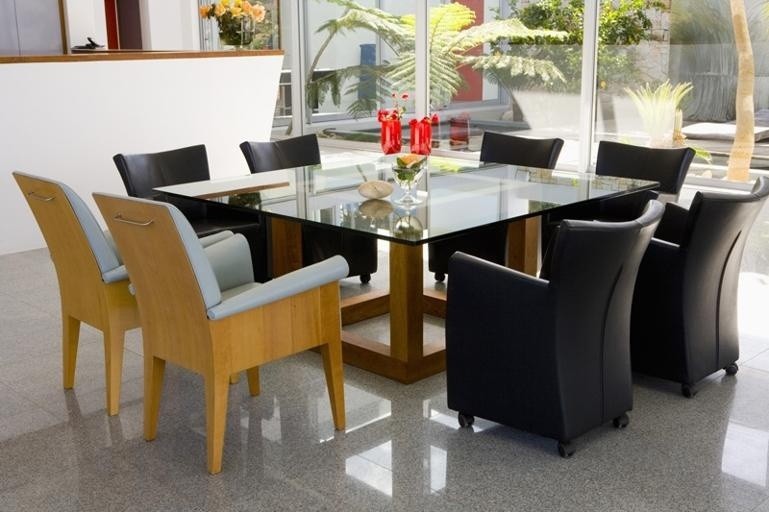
[90,190,348,472]
[427,131,562,283]
[540,139,695,264]
[11,170,240,415]
[240,132,379,283]
[113,142,261,277]
[443,199,666,457]
[612,174,769,401]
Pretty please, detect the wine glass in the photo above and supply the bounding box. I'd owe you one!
[390,167,426,205]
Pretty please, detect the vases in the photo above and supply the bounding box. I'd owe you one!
[378,118,401,154]
[409,124,431,155]
[214,11,254,50]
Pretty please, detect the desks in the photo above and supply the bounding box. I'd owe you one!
[152,149,660,387]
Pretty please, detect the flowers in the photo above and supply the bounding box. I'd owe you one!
[198,1,266,43]
[408,113,439,129]
[375,92,407,121]
